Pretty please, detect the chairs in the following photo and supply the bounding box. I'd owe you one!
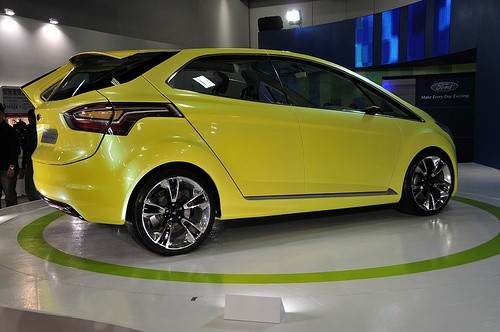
[240,69,276,103]
[208,71,229,95]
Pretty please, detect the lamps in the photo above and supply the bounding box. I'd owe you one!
[286,10,302,27]
[4,8,15,16]
[49,18,59,25]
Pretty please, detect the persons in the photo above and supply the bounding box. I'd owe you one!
[0,102,19,209]
[22,109,44,201]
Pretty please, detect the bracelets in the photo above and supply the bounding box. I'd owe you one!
[9,165,15,170]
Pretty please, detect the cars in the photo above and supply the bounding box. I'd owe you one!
[20,46,457,256]
